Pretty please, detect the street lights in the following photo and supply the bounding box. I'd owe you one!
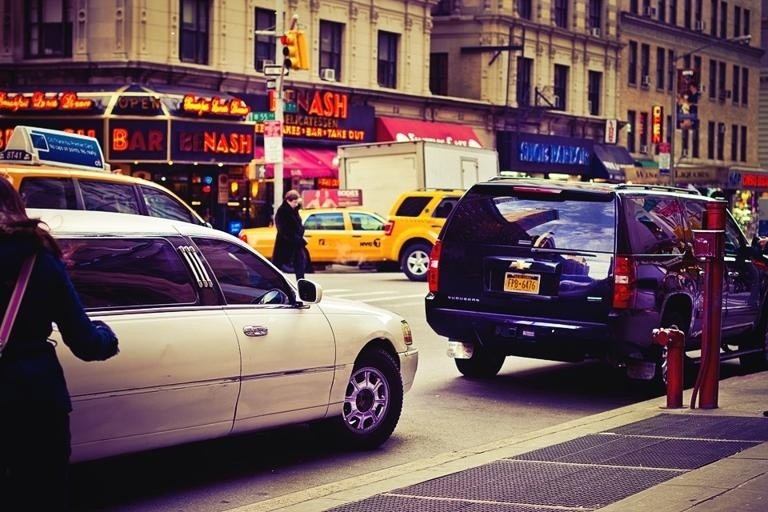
[670,34,752,187]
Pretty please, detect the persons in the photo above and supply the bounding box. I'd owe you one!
[0,178,119,512]
[272,189,315,280]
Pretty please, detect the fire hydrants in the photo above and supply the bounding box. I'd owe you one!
[651,324,686,408]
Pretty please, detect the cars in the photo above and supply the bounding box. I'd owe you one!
[25,208,420,468]
[425,176,768,392]
[238,207,388,273]
[0,124,214,229]
[381,188,467,282]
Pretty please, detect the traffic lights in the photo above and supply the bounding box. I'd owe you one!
[281,31,310,71]
